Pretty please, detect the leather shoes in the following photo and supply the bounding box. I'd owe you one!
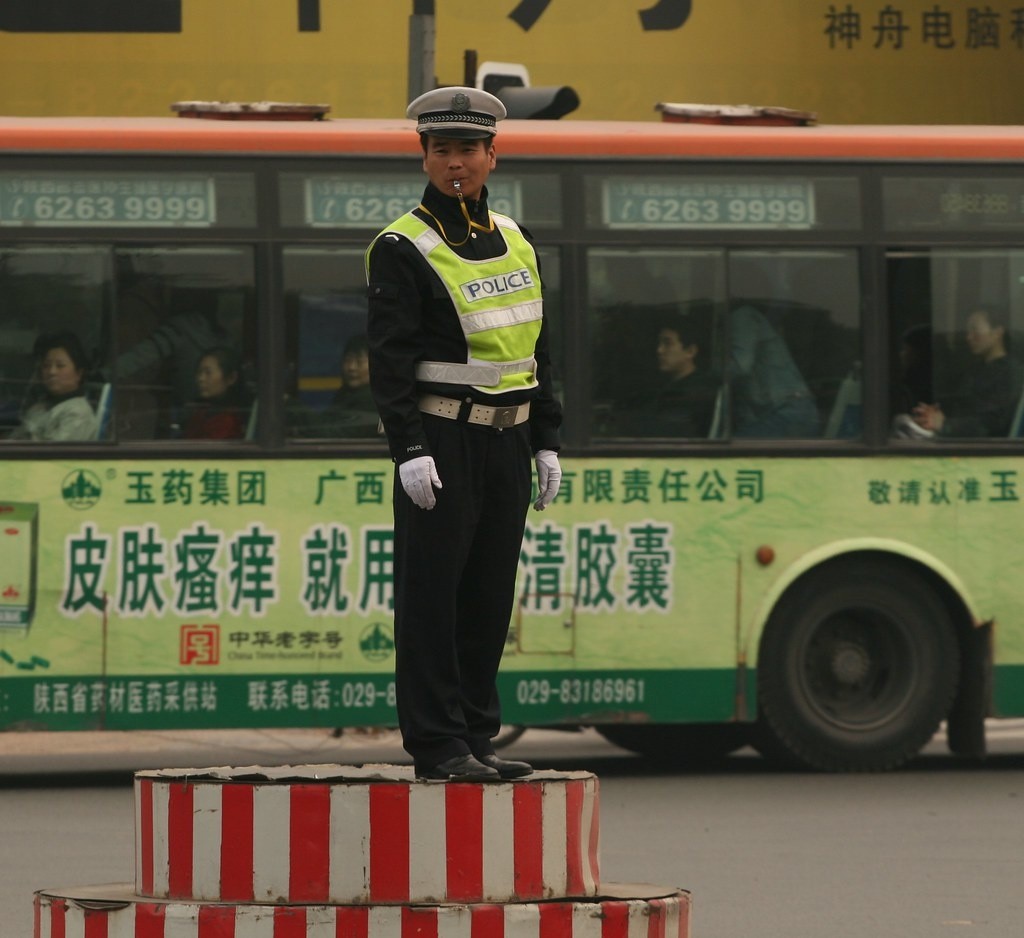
[415,753,502,781]
[474,752,533,778]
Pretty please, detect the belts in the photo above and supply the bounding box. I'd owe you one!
[418,391,530,428]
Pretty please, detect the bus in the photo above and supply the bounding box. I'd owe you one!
[0,99,1024,775]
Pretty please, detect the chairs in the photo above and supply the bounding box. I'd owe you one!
[91,373,863,443]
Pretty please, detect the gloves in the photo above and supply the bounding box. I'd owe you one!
[399,456,442,510]
[533,449,562,512]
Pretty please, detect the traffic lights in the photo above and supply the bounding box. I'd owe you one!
[475,62,582,124]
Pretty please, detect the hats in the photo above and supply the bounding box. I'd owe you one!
[407,86,506,139]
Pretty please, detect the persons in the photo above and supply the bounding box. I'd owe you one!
[181,346,242,439]
[364,86,564,781]
[615,292,1024,449]
[6,323,99,443]
[289,324,376,439]
